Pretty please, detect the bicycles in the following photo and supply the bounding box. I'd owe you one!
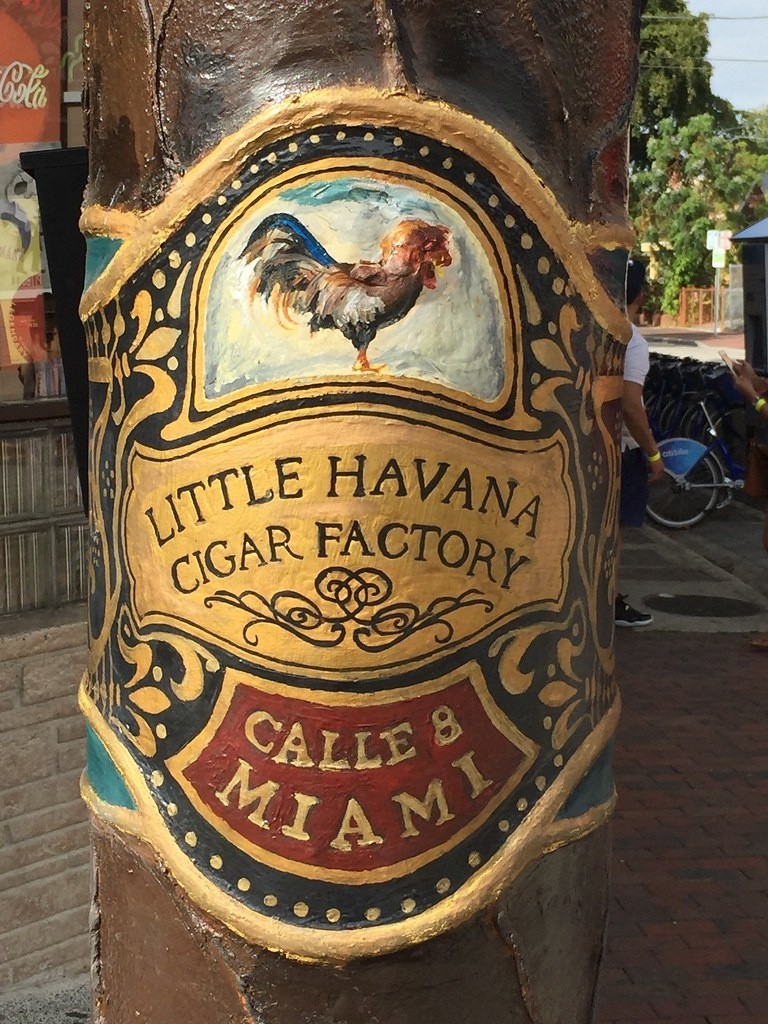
[641,352,751,529]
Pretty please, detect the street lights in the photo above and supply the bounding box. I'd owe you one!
[713,230,732,339]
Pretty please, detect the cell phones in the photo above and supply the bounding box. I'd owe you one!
[719,350,737,376]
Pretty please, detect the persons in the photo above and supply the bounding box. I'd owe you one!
[727,359,768,418]
[615,259,664,627]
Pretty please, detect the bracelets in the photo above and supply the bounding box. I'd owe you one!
[752,396,759,406]
[646,451,661,462]
[755,398,766,411]
